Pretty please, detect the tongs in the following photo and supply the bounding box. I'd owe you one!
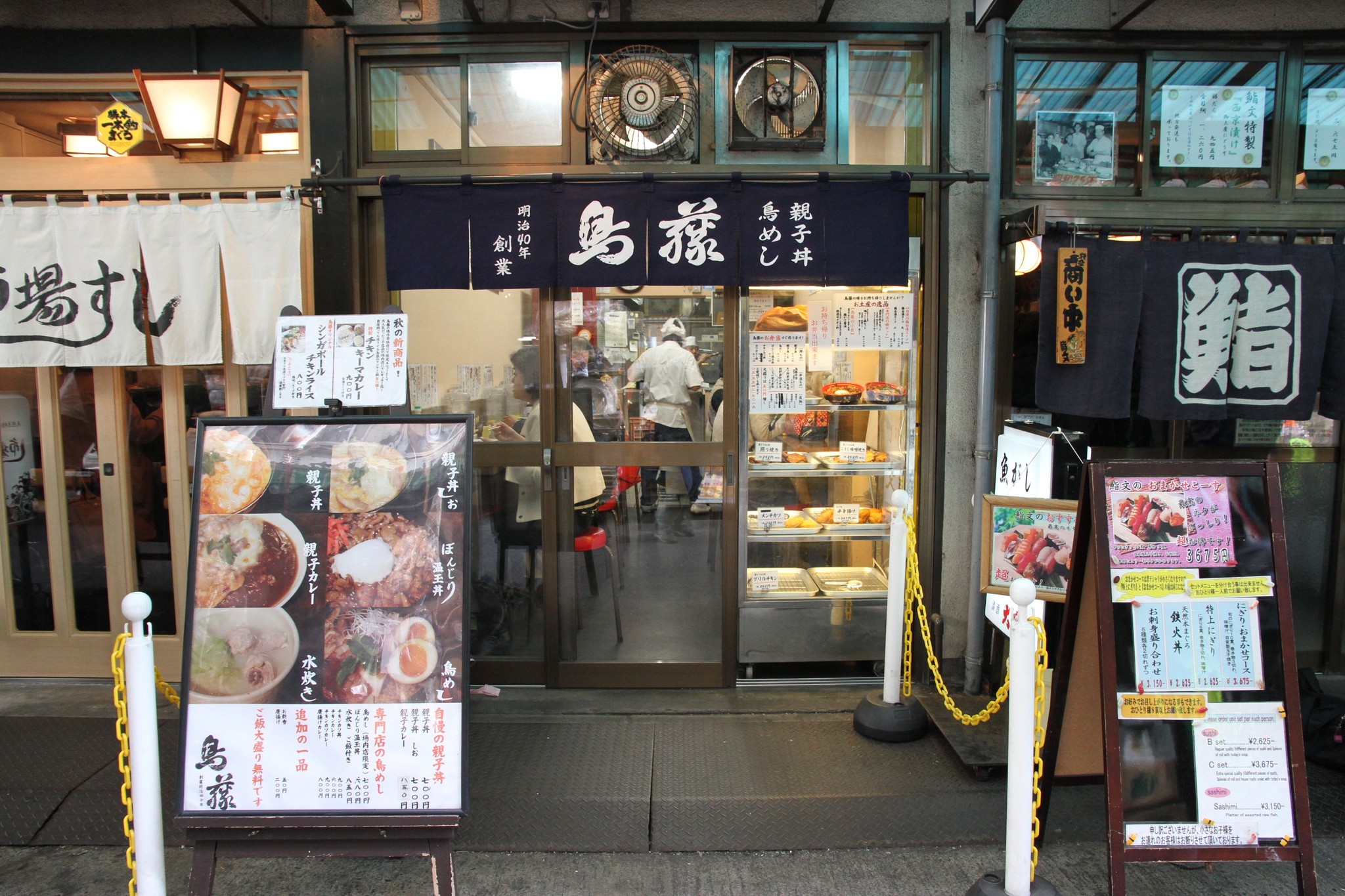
[825,580,862,588]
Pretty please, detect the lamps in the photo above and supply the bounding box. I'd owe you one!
[133,67,249,152]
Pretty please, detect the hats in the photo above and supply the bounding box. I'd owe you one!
[660,318,685,338]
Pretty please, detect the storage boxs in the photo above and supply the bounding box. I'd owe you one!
[630,416,655,442]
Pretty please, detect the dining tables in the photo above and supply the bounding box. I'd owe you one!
[472,439,504,596]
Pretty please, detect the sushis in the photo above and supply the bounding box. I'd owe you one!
[1118,494,1185,541]
[1001,528,1072,582]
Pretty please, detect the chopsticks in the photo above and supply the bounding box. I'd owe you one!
[488,425,500,430]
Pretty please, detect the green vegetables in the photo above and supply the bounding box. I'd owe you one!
[207,535,238,565]
[202,450,226,475]
[882,385,901,394]
[283,327,300,338]
[348,462,369,487]
[336,634,382,687]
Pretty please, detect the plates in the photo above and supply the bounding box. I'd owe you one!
[473,439,498,442]
[337,325,356,347]
[994,524,1073,590]
[1112,493,1187,543]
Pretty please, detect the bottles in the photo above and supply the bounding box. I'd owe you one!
[414,406,422,415]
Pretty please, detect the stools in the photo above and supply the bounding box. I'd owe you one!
[522,485,639,660]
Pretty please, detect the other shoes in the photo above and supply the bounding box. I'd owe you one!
[691,502,710,514]
[641,501,658,512]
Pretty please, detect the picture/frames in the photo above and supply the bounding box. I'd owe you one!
[979,494,1078,604]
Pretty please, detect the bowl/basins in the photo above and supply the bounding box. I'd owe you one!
[354,324,365,336]
[200,425,276,514]
[188,607,300,704]
[365,425,417,512]
[473,434,477,439]
[865,382,907,404]
[401,510,448,608]
[821,382,864,404]
[398,611,445,703]
[198,514,307,607]
[129,383,201,419]
[354,335,363,347]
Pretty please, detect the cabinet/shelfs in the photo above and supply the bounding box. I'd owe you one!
[740,236,920,669]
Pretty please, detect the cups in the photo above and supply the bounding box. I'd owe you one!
[205,374,226,411]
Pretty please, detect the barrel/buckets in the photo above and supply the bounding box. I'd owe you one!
[443,384,470,414]
[483,386,507,423]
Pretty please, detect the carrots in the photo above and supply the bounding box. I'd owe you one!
[326,515,350,554]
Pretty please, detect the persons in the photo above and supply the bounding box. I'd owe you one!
[712,400,818,570]
[58,368,165,472]
[682,336,711,368]
[627,317,711,512]
[705,355,723,440]
[490,347,607,542]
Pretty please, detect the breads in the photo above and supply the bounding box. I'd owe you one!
[817,508,884,524]
[785,517,816,528]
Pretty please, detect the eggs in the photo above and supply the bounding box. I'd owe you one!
[331,538,395,584]
[834,390,850,395]
[387,638,438,684]
[390,616,436,652]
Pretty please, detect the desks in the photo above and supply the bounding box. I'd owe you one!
[623,387,705,477]
